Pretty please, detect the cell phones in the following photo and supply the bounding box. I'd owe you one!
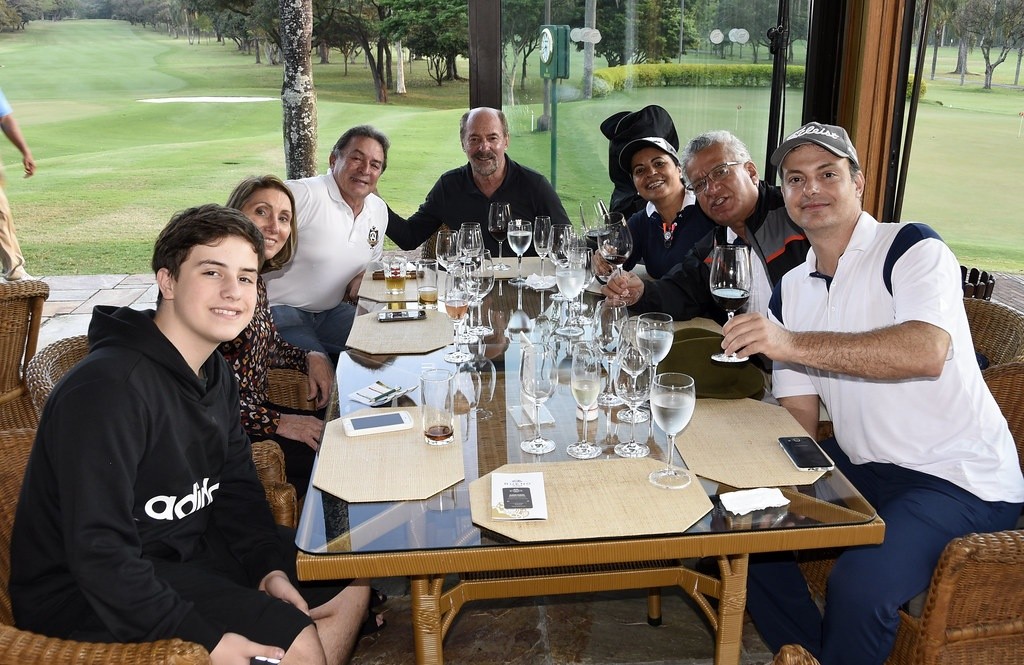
[777,437,834,472]
[342,411,414,436]
[377,310,427,322]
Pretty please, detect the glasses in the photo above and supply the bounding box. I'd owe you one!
[686,160,748,195]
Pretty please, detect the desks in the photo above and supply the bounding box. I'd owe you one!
[293,256,886,665]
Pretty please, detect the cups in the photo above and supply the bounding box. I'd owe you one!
[382,255,406,294]
[415,258,438,311]
[420,370,454,444]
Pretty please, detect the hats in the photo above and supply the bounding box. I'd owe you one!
[770,122,859,185]
[618,135,684,178]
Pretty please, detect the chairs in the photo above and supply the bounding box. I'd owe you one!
[742,298,1024,664]
[0,281,319,665]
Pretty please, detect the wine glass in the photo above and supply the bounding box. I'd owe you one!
[520,344,556,454]
[649,373,696,489]
[617,320,652,424]
[566,342,602,459]
[546,224,593,336]
[597,212,632,275]
[436,221,494,363]
[487,203,514,271]
[507,219,533,286]
[635,312,674,390]
[532,215,555,289]
[614,346,650,457]
[709,245,753,362]
[591,299,629,406]
[506,283,552,342]
[579,198,611,264]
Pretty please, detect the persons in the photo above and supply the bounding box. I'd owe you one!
[3,204,371,665]
[373,107,574,258]
[590,137,719,278]
[265,124,389,369]
[601,130,811,320]
[720,121,1024,665]
[0,88,46,282]
[218,176,388,638]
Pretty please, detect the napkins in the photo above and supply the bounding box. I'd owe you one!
[525,273,556,289]
[719,488,790,516]
